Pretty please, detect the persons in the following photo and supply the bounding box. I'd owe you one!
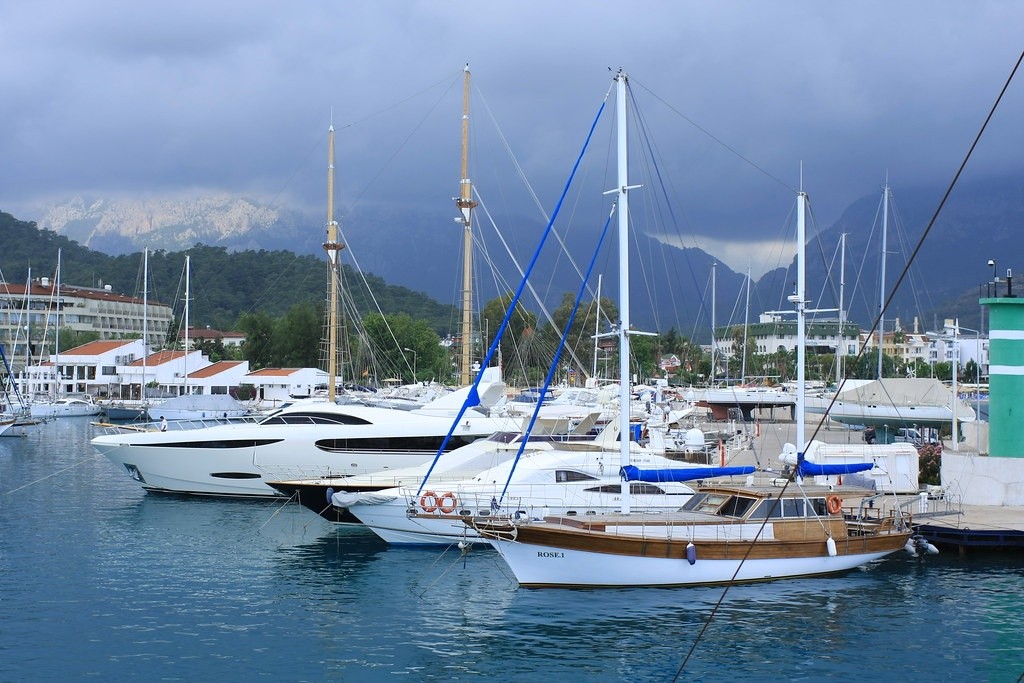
[160,416,168,431]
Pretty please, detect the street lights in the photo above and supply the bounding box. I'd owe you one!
[404,347,416,385]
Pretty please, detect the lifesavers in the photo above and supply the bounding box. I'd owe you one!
[421,490,439,512]
[437,492,459,514]
[828,495,844,515]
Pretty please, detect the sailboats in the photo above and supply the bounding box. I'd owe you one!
[464,68,915,589]
[89,65,652,521]
[0,246,254,437]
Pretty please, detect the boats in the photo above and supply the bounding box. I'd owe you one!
[331,451,698,543]
[335,377,649,430]
